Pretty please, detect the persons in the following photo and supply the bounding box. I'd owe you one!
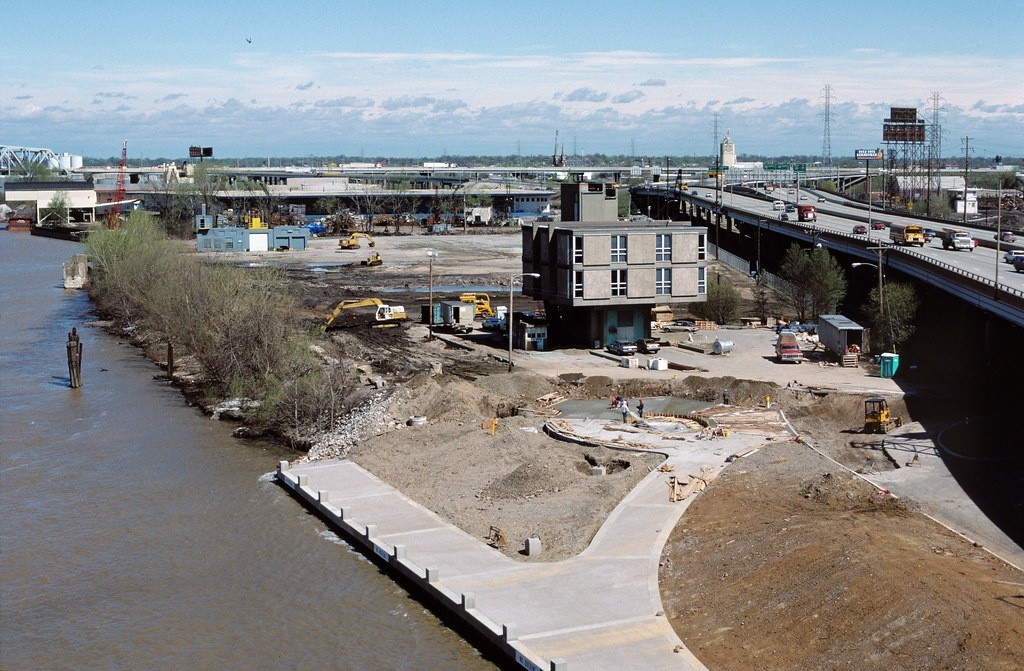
[723,389,731,404]
[612,394,629,423]
[636,399,644,418]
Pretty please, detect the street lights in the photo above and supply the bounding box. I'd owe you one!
[427,249,439,338]
[508,273,541,365]
[852,262,885,351]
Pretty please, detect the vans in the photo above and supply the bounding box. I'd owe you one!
[924,228,936,242]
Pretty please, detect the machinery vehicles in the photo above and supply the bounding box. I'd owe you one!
[319,296,408,332]
[459,293,496,318]
[103,162,125,228]
[337,233,383,267]
[863,397,903,434]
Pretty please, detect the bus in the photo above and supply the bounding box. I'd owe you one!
[889,222,925,248]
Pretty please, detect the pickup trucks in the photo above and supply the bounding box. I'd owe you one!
[773,342,803,363]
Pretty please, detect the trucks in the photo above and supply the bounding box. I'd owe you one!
[798,203,818,222]
[942,227,975,252]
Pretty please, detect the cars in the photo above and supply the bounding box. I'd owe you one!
[606,338,662,356]
[774,201,796,221]
[662,320,700,332]
[692,191,713,197]
[852,225,867,235]
[788,189,826,203]
[1003,250,1024,272]
[481,318,503,330]
[871,220,885,229]
[993,231,1018,243]
